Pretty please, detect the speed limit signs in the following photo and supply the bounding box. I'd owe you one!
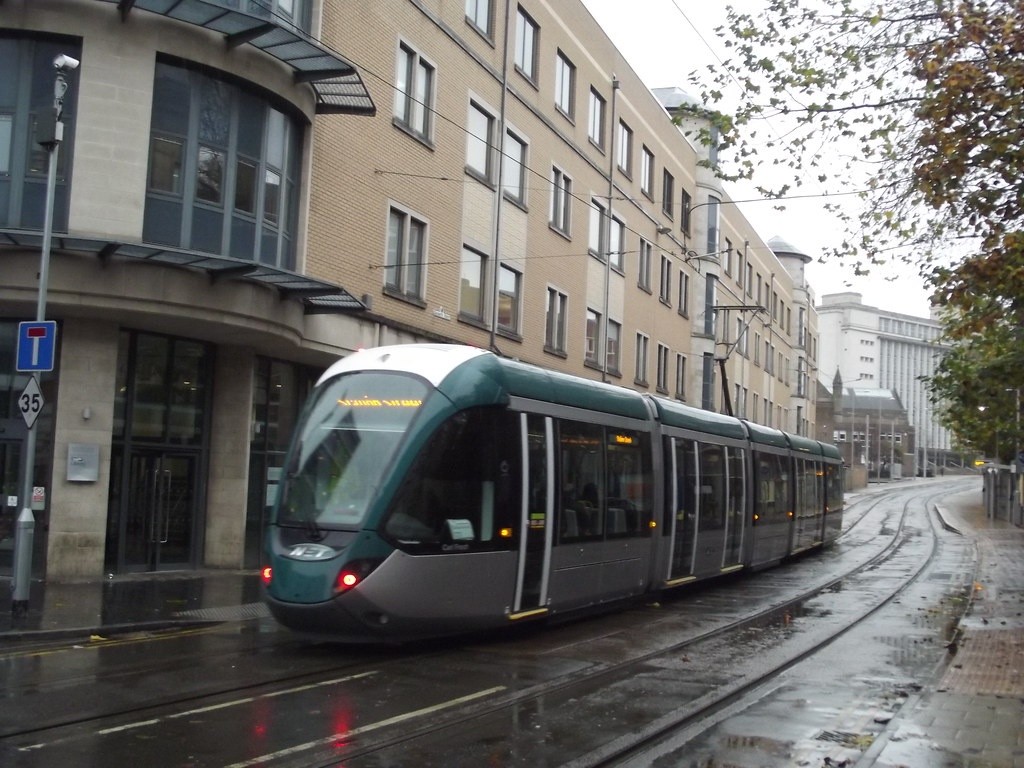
[17,374,47,429]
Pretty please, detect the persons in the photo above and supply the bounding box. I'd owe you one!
[531,465,573,544]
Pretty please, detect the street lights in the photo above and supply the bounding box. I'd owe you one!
[977,404,999,461]
[1004,387,1021,456]
[877,396,896,479]
[890,411,908,480]
[849,389,871,469]
[8,51,82,615]
[923,401,947,477]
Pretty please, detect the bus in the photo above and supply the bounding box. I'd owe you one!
[262,302,845,643]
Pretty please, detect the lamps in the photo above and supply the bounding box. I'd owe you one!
[656,224,672,234]
